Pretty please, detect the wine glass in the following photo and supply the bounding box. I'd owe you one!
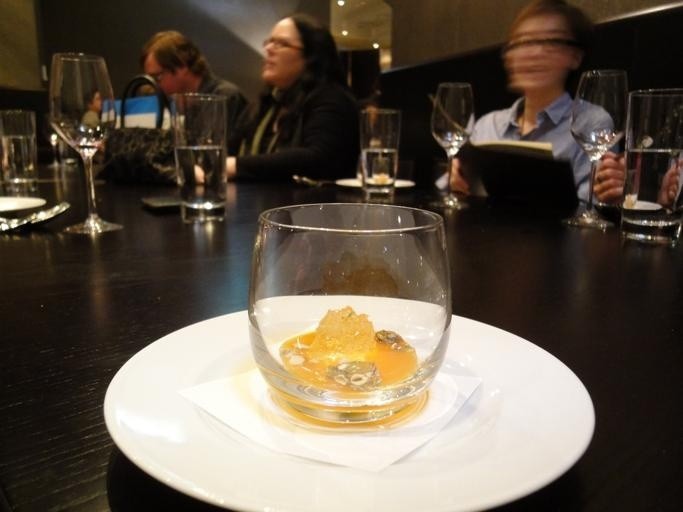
[562,70,629,229]
[47,50,129,235]
[430,82,476,211]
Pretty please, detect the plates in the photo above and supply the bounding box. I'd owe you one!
[102,293,598,511]
[0,196,44,219]
[616,199,663,214]
[333,178,417,190]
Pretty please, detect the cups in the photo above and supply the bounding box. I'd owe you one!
[359,109,402,197]
[1,108,40,197]
[172,91,229,225]
[619,86,683,247]
[246,202,455,425]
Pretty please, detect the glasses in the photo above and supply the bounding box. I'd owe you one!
[263,37,304,53]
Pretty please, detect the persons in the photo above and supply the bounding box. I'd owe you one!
[593,149,626,206]
[435,0,618,209]
[192,13,359,188]
[142,30,252,159]
[657,156,683,210]
[80,88,102,127]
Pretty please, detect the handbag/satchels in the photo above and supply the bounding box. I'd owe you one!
[97,74,183,188]
